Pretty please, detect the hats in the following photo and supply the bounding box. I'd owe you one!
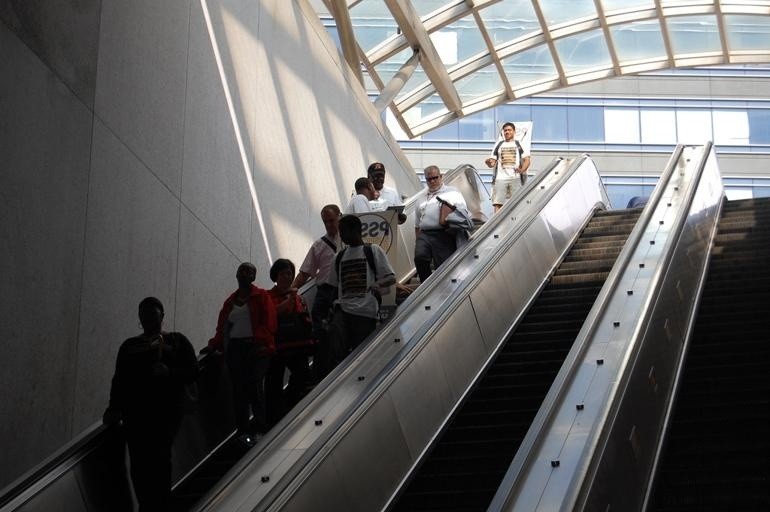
[368,163,385,176]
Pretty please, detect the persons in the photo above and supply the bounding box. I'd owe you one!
[101,296,200,511]
[317,212,397,383]
[206,261,280,461]
[290,203,417,331]
[366,162,401,213]
[262,258,316,430]
[485,121,531,216]
[343,177,378,215]
[412,166,469,285]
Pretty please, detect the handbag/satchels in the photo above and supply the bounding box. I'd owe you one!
[172,382,199,418]
[435,196,456,225]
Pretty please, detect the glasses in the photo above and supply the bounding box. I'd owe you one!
[426,176,439,181]
